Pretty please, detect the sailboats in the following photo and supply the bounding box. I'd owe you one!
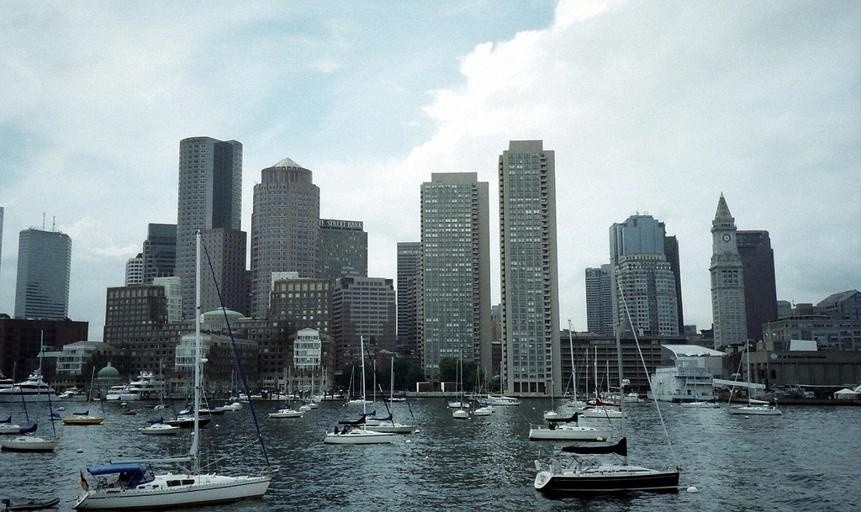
[268,336,417,444]
[529,255,681,495]
[448,350,520,419]
[647,339,783,416]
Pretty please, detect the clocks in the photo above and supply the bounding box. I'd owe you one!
[722,234,732,242]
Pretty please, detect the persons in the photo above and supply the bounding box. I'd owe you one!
[771,399,776,407]
[334,426,347,434]
[549,422,559,429]
